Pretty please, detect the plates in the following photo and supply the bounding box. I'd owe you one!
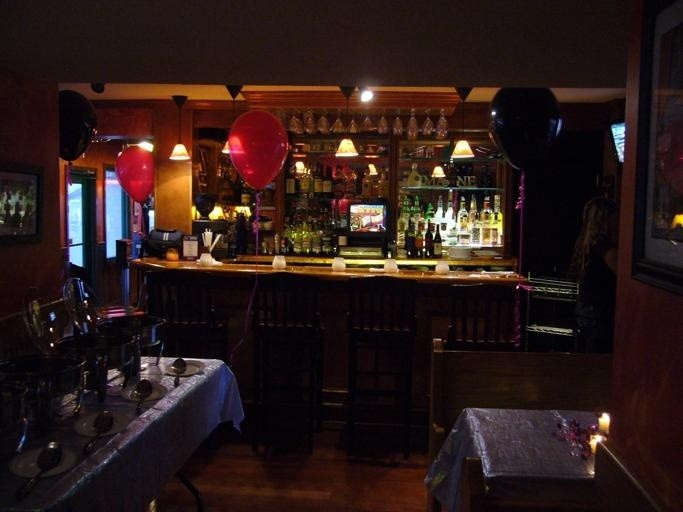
[118,380,170,402]
[162,358,204,379]
[71,408,129,435]
[8,445,76,476]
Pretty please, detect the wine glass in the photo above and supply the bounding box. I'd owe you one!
[278,103,449,137]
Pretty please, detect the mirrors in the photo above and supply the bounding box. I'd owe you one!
[395,140,504,217]
[284,135,394,224]
[193,128,254,220]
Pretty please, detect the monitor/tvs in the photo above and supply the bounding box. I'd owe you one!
[347,202,388,245]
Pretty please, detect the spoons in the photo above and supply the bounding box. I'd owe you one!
[12,438,63,503]
[134,379,149,412]
[83,409,115,455]
[167,357,187,390]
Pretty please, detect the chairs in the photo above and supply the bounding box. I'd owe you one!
[145,269,232,354]
[449,282,527,352]
[342,276,430,444]
[252,273,322,454]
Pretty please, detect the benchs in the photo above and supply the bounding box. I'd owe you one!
[426,337,612,512]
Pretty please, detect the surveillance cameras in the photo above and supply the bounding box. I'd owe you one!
[90,82,108,94]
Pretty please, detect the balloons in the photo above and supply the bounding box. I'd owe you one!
[114,146,155,203]
[489,88,564,173]
[227,109,289,191]
[58,90,97,162]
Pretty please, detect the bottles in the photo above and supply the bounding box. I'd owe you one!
[285,162,388,197]
[397,194,503,258]
[260,206,346,258]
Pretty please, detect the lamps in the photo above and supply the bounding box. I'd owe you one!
[334,79,359,158]
[221,85,244,155]
[136,135,155,155]
[450,86,474,159]
[356,78,375,103]
[430,164,449,179]
[168,94,192,161]
[366,162,380,177]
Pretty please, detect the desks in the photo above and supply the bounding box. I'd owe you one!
[423,407,611,512]
[0,355,247,511]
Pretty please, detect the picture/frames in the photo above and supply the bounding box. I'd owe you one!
[628,1,683,298]
[0,161,46,247]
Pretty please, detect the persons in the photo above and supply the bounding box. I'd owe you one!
[569,195,617,353]
